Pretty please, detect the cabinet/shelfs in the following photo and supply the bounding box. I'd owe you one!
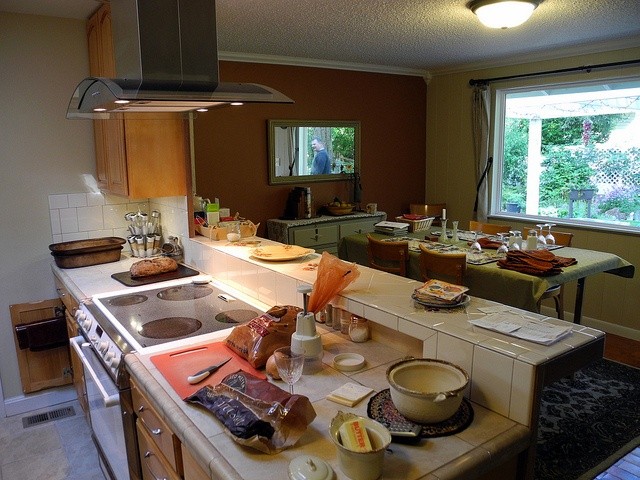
[128,375,181,480]
[266,218,339,258]
[86,3,191,200]
[177,442,211,480]
[339,215,382,241]
[10,275,88,412]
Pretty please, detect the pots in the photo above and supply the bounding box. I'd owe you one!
[385,354,470,423]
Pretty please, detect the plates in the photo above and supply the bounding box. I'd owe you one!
[411,292,471,309]
[250,245,316,262]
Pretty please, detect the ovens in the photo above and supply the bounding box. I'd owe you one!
[68,301,141,480]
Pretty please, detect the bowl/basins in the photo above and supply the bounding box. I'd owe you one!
[328,410,393,480]
[323,203,355,215]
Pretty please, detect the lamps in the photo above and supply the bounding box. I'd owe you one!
[465,1,545,30]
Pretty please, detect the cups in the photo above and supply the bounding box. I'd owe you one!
[366,202,377,215]
[324,304,332,327]
[340,310,351,334]
[331,307,341,330]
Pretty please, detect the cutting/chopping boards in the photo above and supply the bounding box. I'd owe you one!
[149,341,269,402]
[111,264,199,287]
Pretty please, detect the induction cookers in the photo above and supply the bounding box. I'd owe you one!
[88,281,273,357]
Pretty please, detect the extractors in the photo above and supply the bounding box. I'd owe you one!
[66,1,295,120]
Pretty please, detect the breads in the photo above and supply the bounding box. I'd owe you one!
[130,256,180,278]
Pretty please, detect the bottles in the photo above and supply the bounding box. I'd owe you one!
[153,234,161,255]
[193,195,206,236]
[127,236,138,257]
[227,223,242,242]
[314,304,325,324]
[348,315,369,343]
[146,235,154,256]
[509,232,522,249]
[139,214,150,236]
[125,212,134,234]
[526,230,539,251]
[136,236,144,258]
[130,213,140,236]
[149,210,160,234]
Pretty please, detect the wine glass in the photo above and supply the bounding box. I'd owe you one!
[545,222,557,248]
[468,230,483,263]
[275,346,303,398]
[496,232,510,258]
[537,225,548,249]
[509,230,522,253]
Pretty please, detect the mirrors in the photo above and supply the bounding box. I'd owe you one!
[267,118,362,186]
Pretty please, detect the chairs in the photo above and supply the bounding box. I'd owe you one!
[469,220,511,235]
[522,226,573,320]
[366,233,408,277]
[419,244,467,289]
[410,202,447,226]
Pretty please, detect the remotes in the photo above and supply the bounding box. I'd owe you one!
[375,420,423,446]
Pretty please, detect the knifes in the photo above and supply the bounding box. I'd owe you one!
[187,354,234,384]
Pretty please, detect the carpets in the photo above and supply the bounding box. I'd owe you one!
[534,357,640,480]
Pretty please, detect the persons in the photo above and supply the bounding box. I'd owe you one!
[309,136,332,174]
